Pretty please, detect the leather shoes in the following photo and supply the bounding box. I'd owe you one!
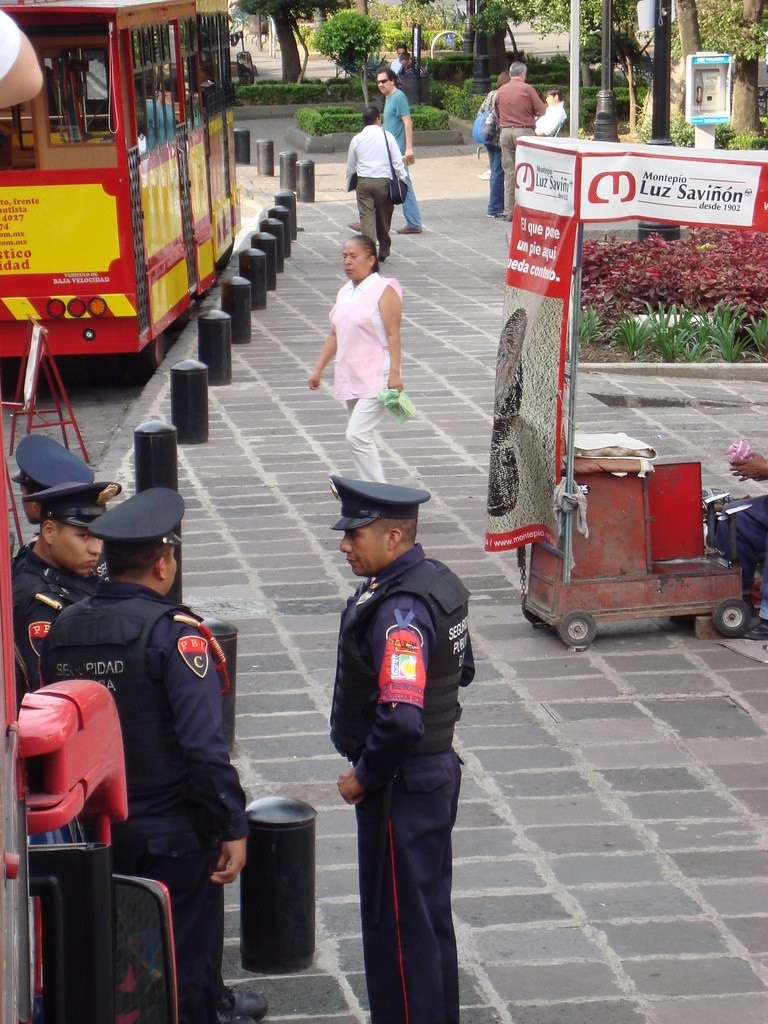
[743,622,768,640]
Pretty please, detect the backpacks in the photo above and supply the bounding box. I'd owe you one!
[471,94,500,146]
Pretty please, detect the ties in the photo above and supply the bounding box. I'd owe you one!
[329,475,431,531]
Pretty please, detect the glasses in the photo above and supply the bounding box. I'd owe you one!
[376,77,393,84]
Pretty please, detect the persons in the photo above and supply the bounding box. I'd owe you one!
[309,235,404,484]
[670,450,768,640]
[42,487,250,1024]
[477,71,511,219]
[346,107,407,262]
[146,81,191,148]
[0,9,44,108]
[7,482,266,1024]
[477,89,567,180]
[10,434,95,559]
[348,68,422,235]
[328,475,476,1024]
[494,62,547,221]
[380,44,428,112]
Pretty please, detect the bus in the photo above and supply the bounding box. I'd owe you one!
[1,434,182,1024]
[0,0,255,366]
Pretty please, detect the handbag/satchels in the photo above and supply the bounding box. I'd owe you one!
[377,387,416,424]
[388,177,408,204]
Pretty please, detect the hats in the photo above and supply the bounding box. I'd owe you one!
[15,435,95,493]
[87,485,185,562]
[23,482,121,528]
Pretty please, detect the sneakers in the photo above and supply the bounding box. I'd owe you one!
[215,1014,257,1024]
[216,984,268,1020]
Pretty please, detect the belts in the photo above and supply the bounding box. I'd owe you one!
[503,124,534,129]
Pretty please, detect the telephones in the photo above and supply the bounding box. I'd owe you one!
[695,69,721,111]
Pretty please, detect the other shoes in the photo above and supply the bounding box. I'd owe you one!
[378,254,386,262]
[397,225,422,233]
[372,260,379,272]
[486,212,503,218]
[502,214,512,222]
[348,222,361,230]
